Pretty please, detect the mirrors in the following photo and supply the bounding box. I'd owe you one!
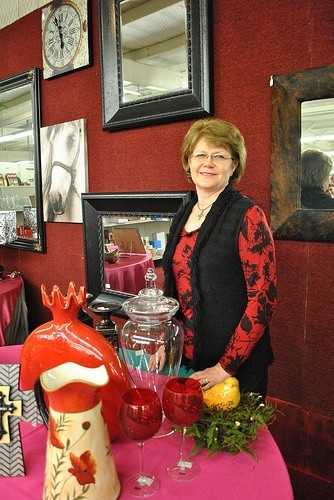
[79,190,194,319]
[96,0,213,130]
[0,68,48,256]
[266,63,334,242]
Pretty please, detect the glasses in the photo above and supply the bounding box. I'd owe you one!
[189,151,234,163]
[328,173,333,179]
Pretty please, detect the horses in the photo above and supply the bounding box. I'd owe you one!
[42,119,82,222]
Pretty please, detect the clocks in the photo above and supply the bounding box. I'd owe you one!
[40,0,93,80]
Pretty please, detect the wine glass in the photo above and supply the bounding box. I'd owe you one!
[162,377,204,482]
[119,387,162,498]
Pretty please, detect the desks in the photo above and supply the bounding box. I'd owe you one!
[0,270,29,347]
[0,344,294,500]
[102,252,156,294]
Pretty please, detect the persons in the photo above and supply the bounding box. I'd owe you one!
[147,117,278,407]
[300,148,334,209]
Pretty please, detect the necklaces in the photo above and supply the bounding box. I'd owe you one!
[195,199,215,222]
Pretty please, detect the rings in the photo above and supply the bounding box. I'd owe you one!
[204,377,209,383]
